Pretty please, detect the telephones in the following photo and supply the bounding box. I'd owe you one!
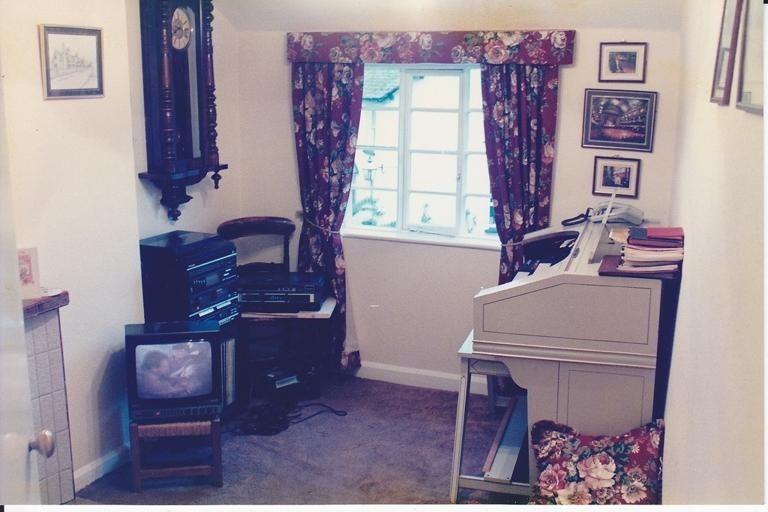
[591,201,644,226]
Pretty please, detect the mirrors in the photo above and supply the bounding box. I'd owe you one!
[709,0,763,116]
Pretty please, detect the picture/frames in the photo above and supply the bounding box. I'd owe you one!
[592,156,642,198]
[580,88,659,153]
[598,42,647,83]
[38,23,104,101]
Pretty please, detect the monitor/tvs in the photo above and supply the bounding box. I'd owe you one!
[124,320,225,422]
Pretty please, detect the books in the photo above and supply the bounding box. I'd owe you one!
[615,226,685,275]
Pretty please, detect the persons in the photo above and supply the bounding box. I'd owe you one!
[137,351,187,396]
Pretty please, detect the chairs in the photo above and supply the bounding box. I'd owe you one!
[216,216,296,274]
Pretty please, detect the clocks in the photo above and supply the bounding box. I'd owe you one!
[137,0,228,221]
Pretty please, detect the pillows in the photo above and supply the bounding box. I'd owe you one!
[530,419,664,504]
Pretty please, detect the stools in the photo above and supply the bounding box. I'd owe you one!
[125,416,223,493]
